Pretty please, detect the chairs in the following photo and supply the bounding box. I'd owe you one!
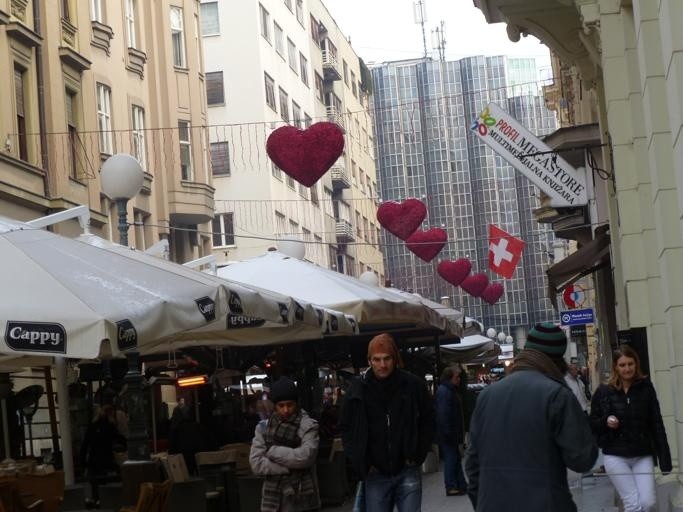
[0,435,345,512]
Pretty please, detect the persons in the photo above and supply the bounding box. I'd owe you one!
[62,321,674,511]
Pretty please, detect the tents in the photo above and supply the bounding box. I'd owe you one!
[23,206,503,456]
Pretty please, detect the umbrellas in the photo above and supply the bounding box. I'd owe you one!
[1,211,230,487]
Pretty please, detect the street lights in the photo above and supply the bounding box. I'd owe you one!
[276,235,307,260]
[358,270,380,287]
[486,327,513,344]
[97,152,147,250]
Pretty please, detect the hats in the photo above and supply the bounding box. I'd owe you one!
[524,322,567,360]
[273,378,296,402]
[368,334,397,359]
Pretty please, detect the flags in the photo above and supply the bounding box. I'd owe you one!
[487,223,525,281]
